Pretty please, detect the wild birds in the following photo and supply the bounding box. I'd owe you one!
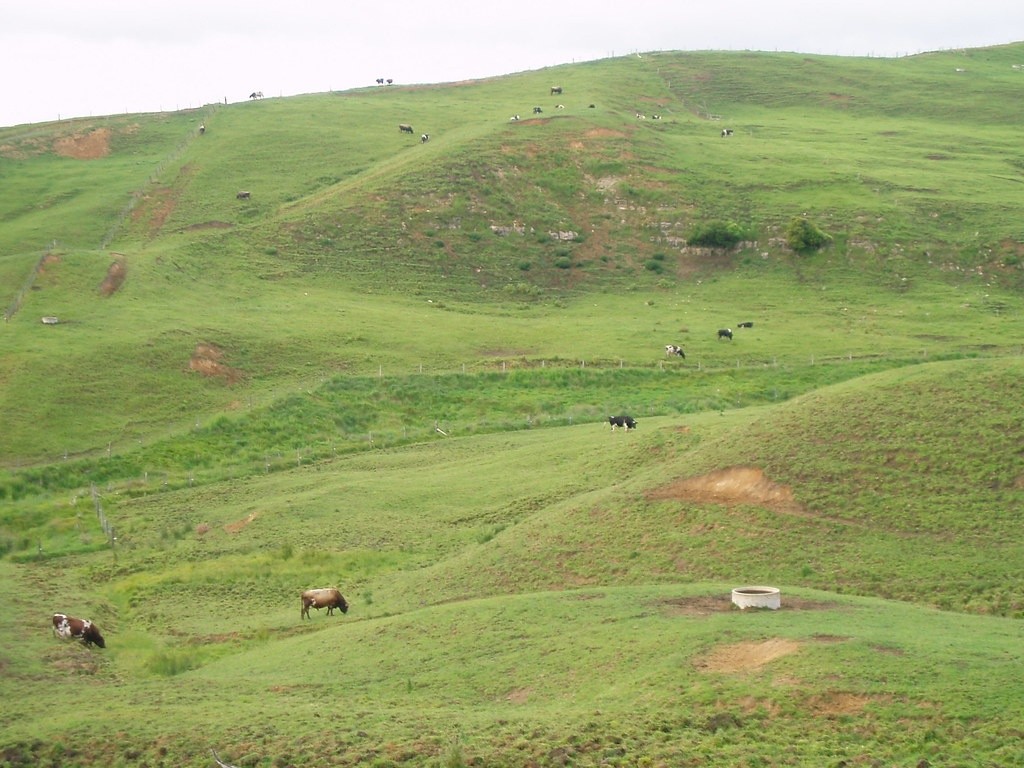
[718,328,732,341]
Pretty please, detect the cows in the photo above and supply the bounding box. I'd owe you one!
[199,123,205,134]
[665,344,686,359]
[510,114,520,121]
[421,133,429,143]
[533,106,542,113]
[636,114,646,120]
[738,322,753,328]
[608,415,638,432]
[301,588,350,619]
[555,105,565,109]
[249,92,264,100]
[652,115,662,119]
[550,86,563,96]
[720,129,734,138]
[376,78,392,85]
[235,191,250,199]
[399,124,414,134]
[52,613,107,649]
